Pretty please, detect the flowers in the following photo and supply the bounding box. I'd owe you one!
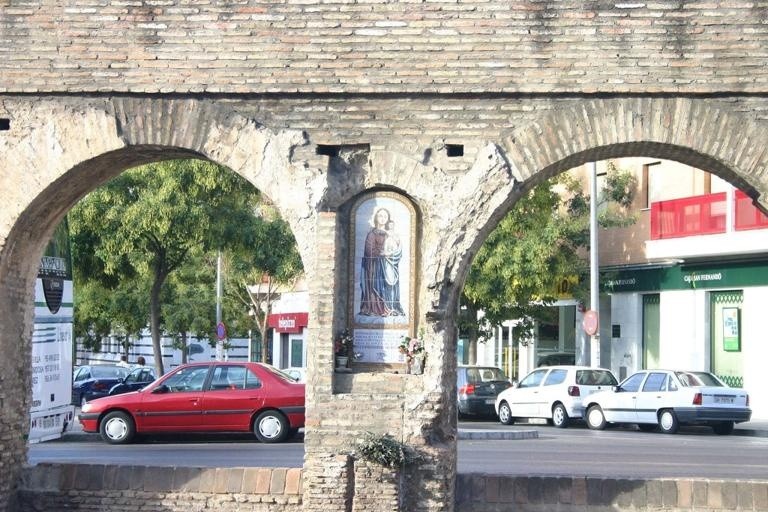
[335,327,363,362]
[397,327,425,357]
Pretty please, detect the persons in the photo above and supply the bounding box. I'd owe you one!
[115,355,129,368]
[358,208,406,317]
[381,219,401,256]
[134,356,146,368]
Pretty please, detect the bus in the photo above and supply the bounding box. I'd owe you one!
[28,214,76,445]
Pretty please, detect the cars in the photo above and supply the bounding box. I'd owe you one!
[582,370,752,434]
[78,360,305,444]
[494,365,618,427]
[73,364,306,406]
[456,365,512,420]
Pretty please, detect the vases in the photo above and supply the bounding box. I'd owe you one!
[335,356,349,368]
[409,356,422,374]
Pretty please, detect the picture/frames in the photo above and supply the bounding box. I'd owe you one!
[722,306,742,352]
